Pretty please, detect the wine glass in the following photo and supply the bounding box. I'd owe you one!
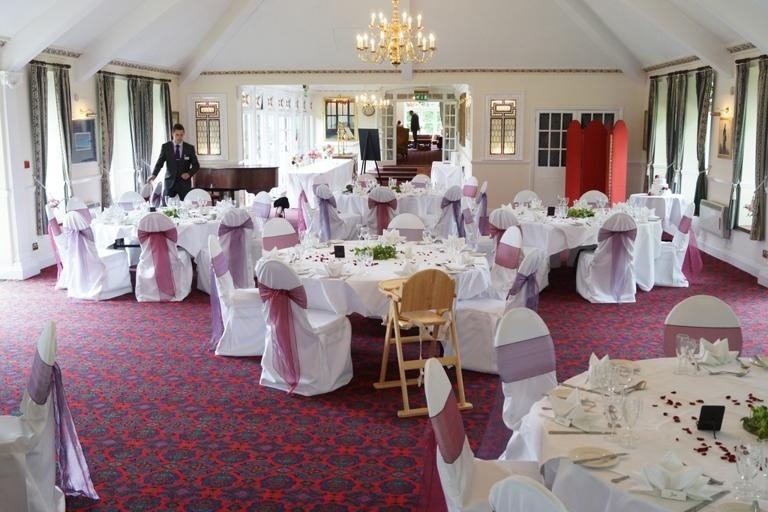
[732,440,766,511]
[299,221,458,272]
[674,334,707,376]
[131,192,237,224]
[346,176,439,191]
[597,359,648,450]
[513,196,584,223]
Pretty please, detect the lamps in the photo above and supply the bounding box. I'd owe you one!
[355,93,377,116]
[357,0,436,68]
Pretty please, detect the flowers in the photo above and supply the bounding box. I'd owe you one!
[309,150,320,159]
[323,144,334,159]
[291,153,304,165]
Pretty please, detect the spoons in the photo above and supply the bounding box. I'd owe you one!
[709,355,768,376]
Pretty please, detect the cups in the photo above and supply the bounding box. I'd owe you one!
[587,200,656,224]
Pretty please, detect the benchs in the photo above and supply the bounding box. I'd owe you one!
[416,135,432,149]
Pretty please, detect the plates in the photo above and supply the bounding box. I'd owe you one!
[567,446,618,469]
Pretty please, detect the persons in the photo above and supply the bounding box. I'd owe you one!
[147,124,199,207]
[409,111,419,149]
[397,121,403,127]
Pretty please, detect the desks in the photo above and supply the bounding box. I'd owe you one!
[490,199,662,293]
[628,192,694,229]
[90,205,232,268]
[265,236,490,319]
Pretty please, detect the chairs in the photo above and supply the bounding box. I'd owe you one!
[194,208,256,296]
[0,319,100,512]
[487,204,523,244]
[575,188,609,209]
[514,188,542,208]
[420,355,546,511]
[374,269,473,418]
[575,212,637,303]
[493,305,559,429]
[650,202,697,288]
[46,188,212,302]
[488,473,567,511]
[445,225,542,376]
[209,175,488,241]
[663,293,742,358]
[256,259,355,396]
[205,235,275,358]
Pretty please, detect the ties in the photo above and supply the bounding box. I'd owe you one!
[176,145,180,159]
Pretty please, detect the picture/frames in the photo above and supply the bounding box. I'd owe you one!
[718,117,732,159]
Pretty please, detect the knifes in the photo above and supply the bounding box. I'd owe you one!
[683,490,731,512]
[571,452,628,464]
[540,379,610,438]
